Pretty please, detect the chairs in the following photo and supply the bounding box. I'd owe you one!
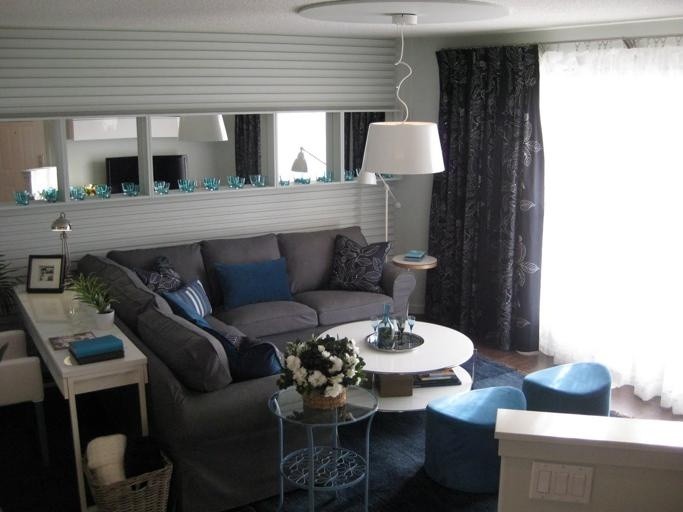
[0,329,50,469]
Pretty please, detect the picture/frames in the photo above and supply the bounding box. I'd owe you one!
[27,255,65,293]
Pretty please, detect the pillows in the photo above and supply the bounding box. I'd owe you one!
[321,234,393,294]
[124,255,293,382]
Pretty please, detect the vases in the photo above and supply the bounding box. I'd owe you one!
[302,386,348,411]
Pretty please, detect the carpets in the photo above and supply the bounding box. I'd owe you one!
[0,350,636,510]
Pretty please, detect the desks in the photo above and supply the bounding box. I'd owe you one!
[493,408,683,512]
[14,280,148,511]
[316,318,475,415]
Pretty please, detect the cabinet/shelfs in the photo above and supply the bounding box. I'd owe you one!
[20,165,59,201]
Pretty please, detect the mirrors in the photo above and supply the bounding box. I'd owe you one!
[0,110,395,201]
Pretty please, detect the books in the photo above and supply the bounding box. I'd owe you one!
[69,348,124,365]
[404,250,426,261]
[69,335,123,359]
[414,369,461,387]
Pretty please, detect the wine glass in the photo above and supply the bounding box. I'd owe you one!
[396,316,417,345]
[369,315,379,336]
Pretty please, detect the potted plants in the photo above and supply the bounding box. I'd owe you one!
[68,271,119,331]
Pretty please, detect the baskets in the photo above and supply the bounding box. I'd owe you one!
[81,445,172,511]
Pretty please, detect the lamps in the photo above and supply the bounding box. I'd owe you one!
[177,112,228,143]
[359,15,446,177]
[51,213,72,279]
[292,147,328,172]
[354,170,402,263]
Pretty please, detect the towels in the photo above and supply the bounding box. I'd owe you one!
[125,437,170,491]
[85,433,127,485]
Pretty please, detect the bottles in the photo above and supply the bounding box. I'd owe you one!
[380,301,394,349]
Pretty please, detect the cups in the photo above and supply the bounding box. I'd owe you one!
[13,169,374,206]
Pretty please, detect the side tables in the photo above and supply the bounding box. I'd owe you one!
[267,383,380,512]
[393,254,439,319]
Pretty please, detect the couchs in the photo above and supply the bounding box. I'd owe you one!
[76,224,416,442]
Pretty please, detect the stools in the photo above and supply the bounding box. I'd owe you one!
[426,386,527,495]
[522,362,611,416]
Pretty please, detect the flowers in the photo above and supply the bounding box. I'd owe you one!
[275,331,366,397]
